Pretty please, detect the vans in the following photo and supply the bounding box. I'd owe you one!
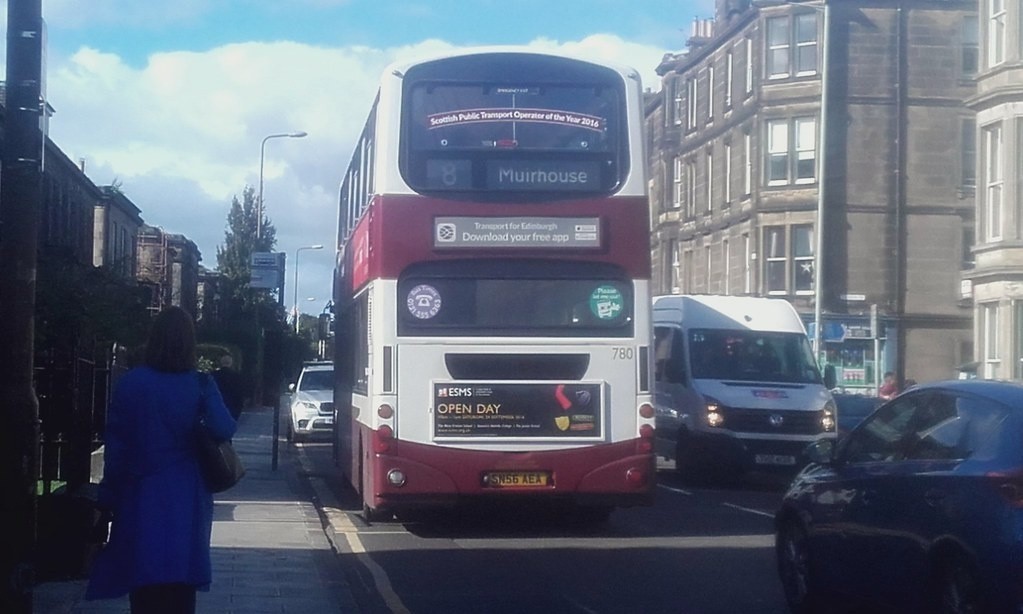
[649,294,839,486]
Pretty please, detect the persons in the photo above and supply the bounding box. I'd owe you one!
[98,305,237,614]
[879,370,917,400]
[212,356,244,422]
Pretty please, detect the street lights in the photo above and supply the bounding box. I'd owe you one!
[295,244,323,336]
[252,131,308,407]
[298,298,314,331]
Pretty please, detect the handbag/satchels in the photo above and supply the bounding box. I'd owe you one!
[193,371,247,496]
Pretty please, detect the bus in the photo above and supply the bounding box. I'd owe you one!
[331,45,656,524]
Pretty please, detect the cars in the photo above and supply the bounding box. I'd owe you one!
[772,380,1023,614]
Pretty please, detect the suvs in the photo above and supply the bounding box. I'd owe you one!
[287,361,334,442]
